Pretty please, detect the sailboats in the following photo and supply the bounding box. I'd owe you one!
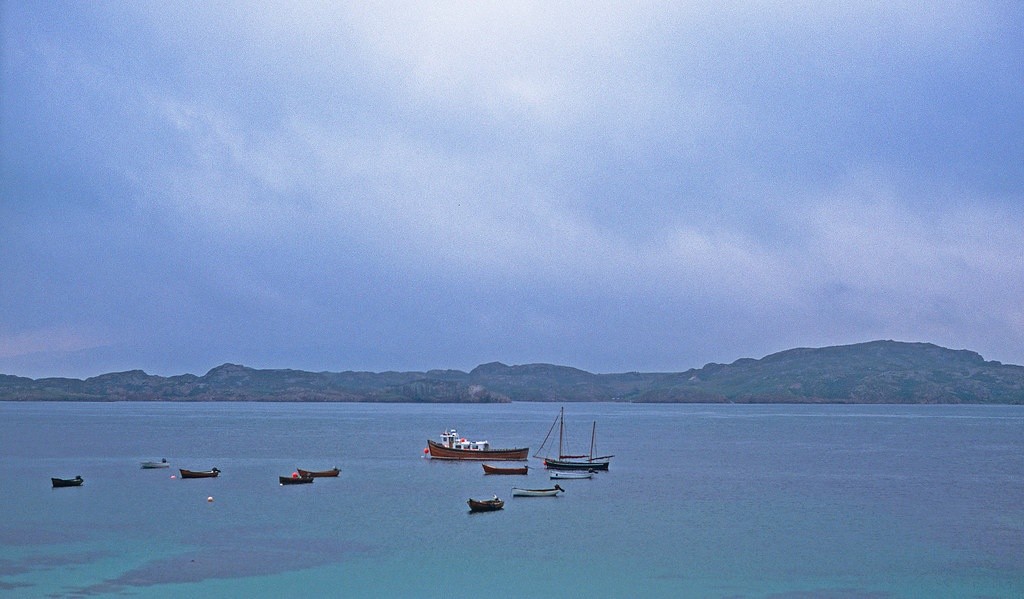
[534,409,614,471]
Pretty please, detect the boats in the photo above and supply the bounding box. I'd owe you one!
[298,468,341,478]
[279,475,314,484]
[512,486,566,497]
[52,476,82,488]
[427,430,529,461]
[551,473,594,481]
[141,459,171,470]
[480,463,527,474]
[178,466,219,478]
[467,496,503,511]
